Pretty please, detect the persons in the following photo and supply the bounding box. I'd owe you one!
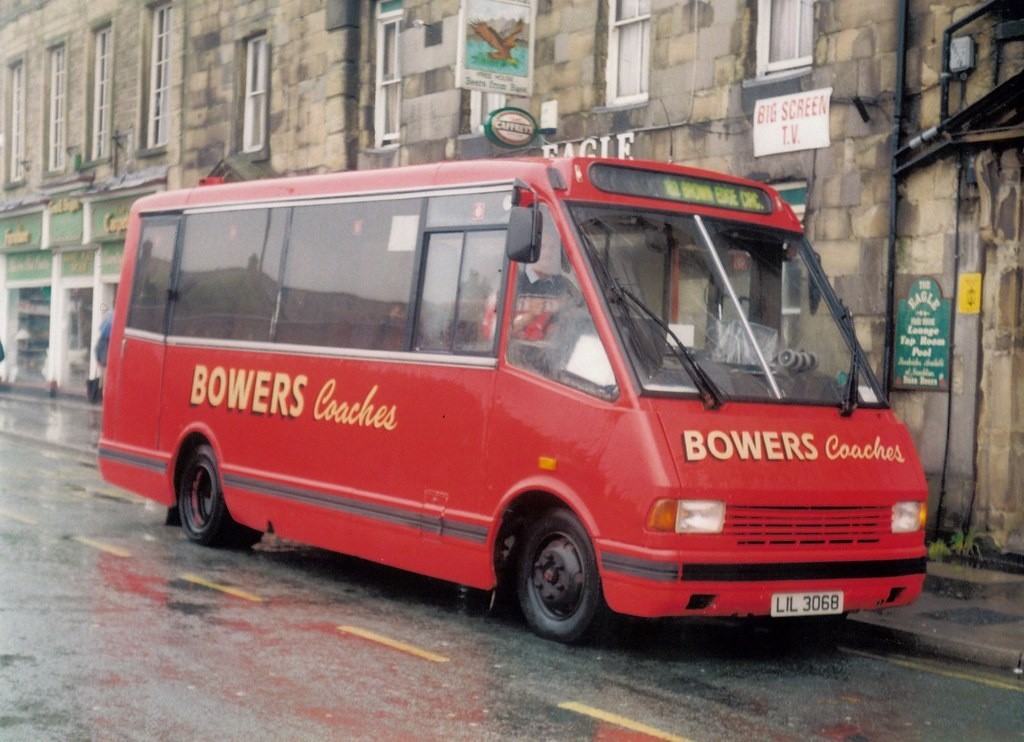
[93,336,109,388]
[479,232,580,344]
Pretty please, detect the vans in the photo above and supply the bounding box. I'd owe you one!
[95,155,927,645]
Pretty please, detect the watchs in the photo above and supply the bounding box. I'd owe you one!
[517,313,525,328]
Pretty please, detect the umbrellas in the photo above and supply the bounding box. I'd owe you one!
[98,312,113,339]
